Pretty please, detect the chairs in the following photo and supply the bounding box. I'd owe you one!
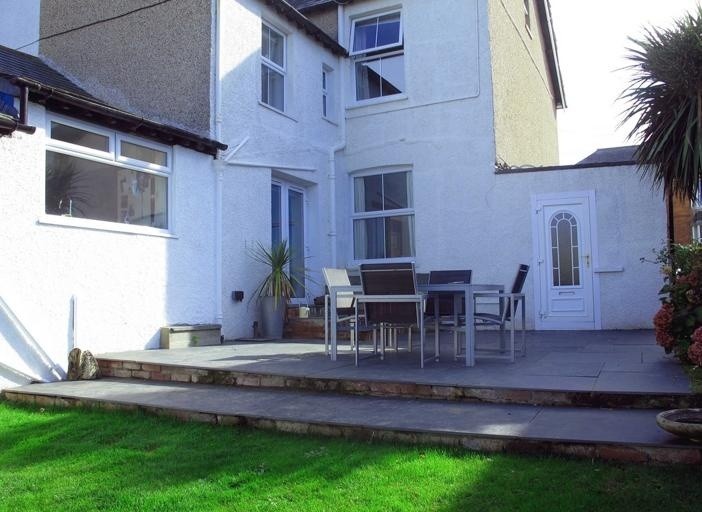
[322,259,540,371]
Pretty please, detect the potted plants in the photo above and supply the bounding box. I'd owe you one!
[249,239,321,345]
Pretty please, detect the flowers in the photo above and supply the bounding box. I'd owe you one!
[640,244,702,370]
